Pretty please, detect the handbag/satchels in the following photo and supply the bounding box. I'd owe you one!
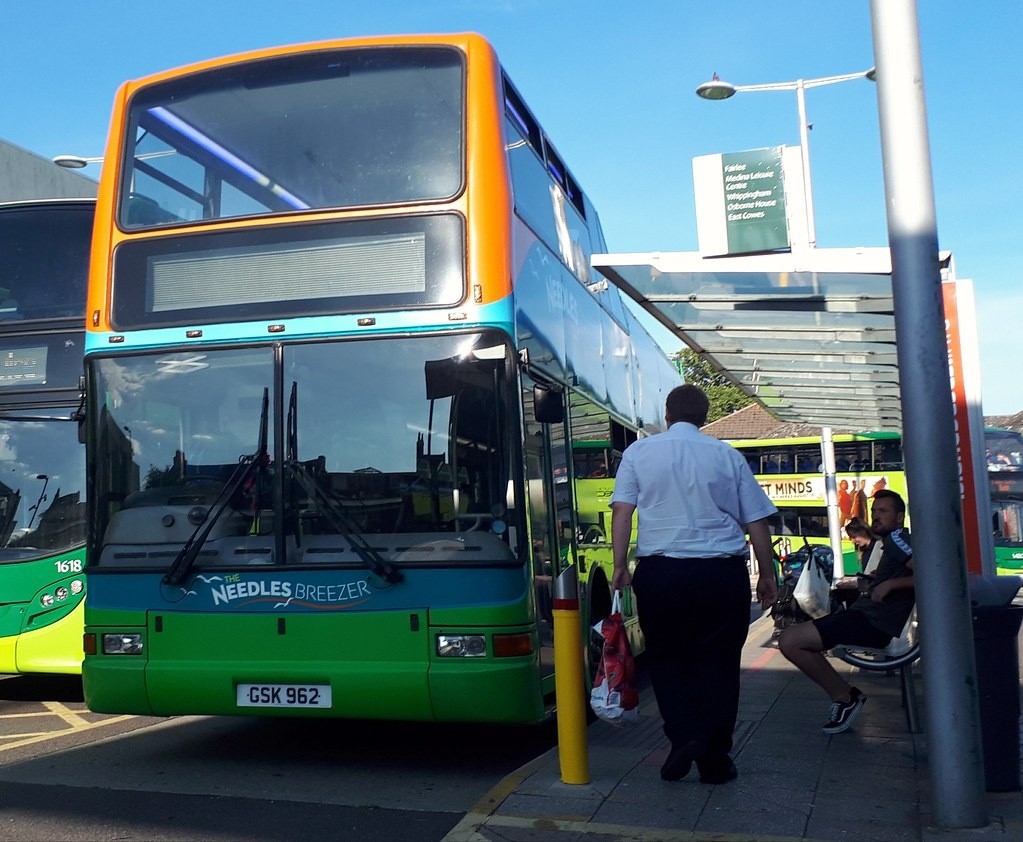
[792,553,832,619]
[590,589,640,728]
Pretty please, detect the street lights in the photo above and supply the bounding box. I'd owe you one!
[696,68,879,328]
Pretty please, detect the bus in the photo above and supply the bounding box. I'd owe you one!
[0,197,210,674]
[723,428,1023,598]
[69,33,687,729]
[406,441,644,622]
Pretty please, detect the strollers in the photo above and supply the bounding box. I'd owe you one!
[771,537,834,643]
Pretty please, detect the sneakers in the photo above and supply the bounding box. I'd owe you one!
[821,686,867,734]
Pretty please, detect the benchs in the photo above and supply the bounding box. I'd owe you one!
[832,601,920,733]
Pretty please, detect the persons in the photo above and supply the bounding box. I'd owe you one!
[778,489,916,735]
[845,516,877,571]
[837,479,875,524]
[608,384,780,784]
[195,436,254,534]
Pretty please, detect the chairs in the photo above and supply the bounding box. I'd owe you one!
[749,458,882,474]
[768,521,829,537]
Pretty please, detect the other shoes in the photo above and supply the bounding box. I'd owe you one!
[695,752,736,785]
[659,739,695,781]
